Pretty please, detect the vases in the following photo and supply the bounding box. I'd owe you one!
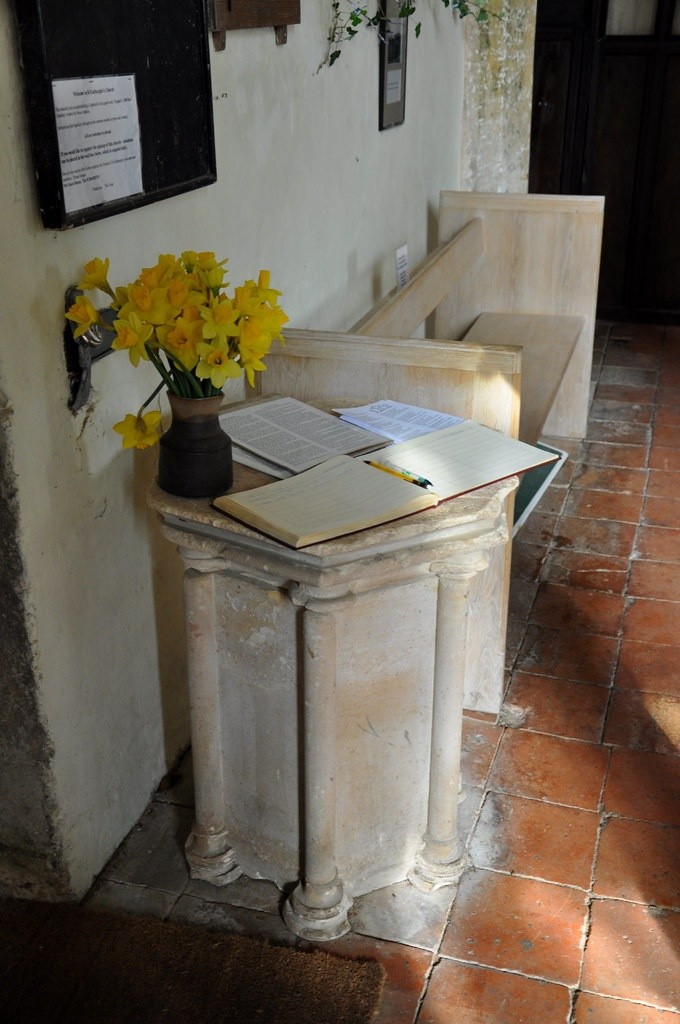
[158,390,234,498]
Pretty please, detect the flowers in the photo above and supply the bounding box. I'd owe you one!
[66,252,289,449]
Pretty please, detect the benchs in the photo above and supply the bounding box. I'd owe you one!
[262,191,606,713]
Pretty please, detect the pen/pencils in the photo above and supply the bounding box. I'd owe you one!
[362,460,434,491]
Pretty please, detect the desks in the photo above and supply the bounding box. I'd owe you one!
[147,397,521,943]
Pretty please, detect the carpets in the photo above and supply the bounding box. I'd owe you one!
[0,893,386,1024]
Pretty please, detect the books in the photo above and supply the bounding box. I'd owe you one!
[210,398,562,551]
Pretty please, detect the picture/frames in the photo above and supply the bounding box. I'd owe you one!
[377,0,409,132]
